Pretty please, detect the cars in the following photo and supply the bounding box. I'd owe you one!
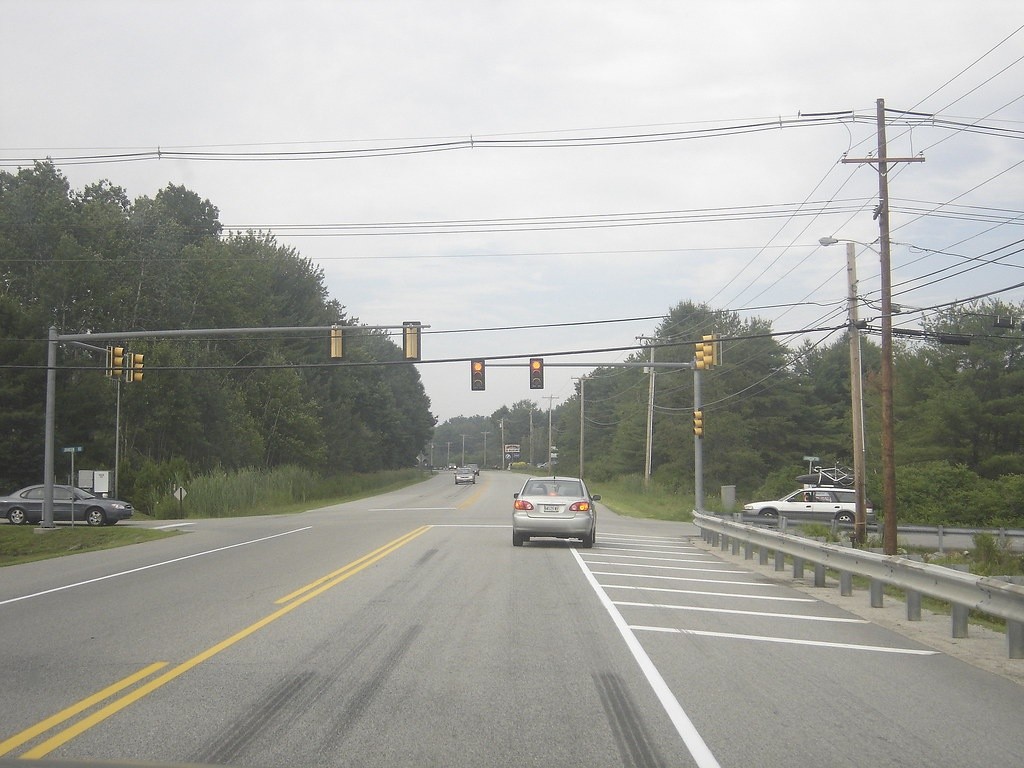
[507,462,549,471]
[0,484,134,526]
[454,468,475,484]
[467,464,480,476]
[449,463,457,470]
[512,474,601,548]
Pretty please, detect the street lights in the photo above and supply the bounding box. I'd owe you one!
[819,236,897,556]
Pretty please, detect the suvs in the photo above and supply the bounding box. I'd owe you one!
[742,465,876,533]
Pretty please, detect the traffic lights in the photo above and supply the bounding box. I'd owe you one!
[131,353,144,382]
[702,335,717,365]
[696,341,709,369]
[530,358,543,389]
[471,359,485,391]
[111,346,124,376]
[693,407,705,439]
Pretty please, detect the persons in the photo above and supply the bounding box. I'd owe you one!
[804,493,810,501]
[537,484,547,494]
